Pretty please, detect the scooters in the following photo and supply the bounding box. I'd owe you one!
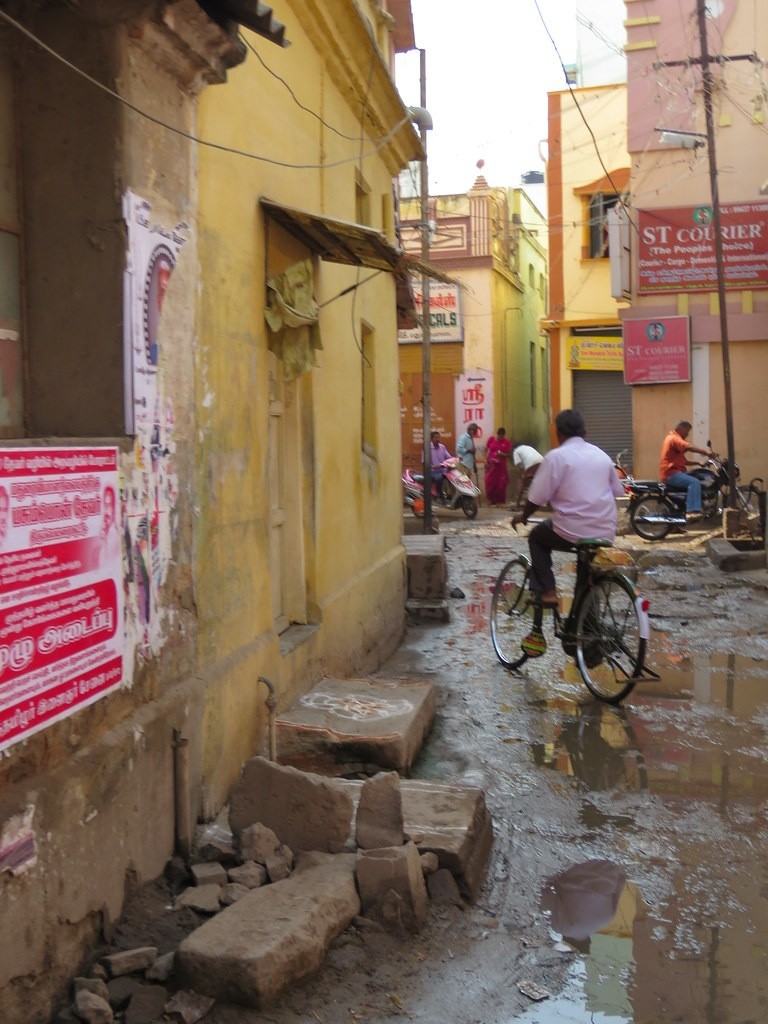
[401,455,482,519]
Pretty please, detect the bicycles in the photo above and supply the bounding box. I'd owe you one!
[489,517,662,704]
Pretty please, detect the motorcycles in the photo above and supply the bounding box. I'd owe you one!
[615,440,764,542]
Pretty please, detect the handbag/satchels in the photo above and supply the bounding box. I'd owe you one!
[593,546,635,565]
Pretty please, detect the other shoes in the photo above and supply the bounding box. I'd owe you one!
[686,512,703,522]
[541,590,560,609]
[445,496,453,501]
[506,507,520,511]
[669,528,687,534]
[439,495,446,503]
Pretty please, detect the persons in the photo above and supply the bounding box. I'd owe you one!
[423,423,553,511]
[512,408,624,669]
[660,418,713,521]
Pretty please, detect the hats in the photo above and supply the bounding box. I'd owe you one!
[467,423,477,432]
